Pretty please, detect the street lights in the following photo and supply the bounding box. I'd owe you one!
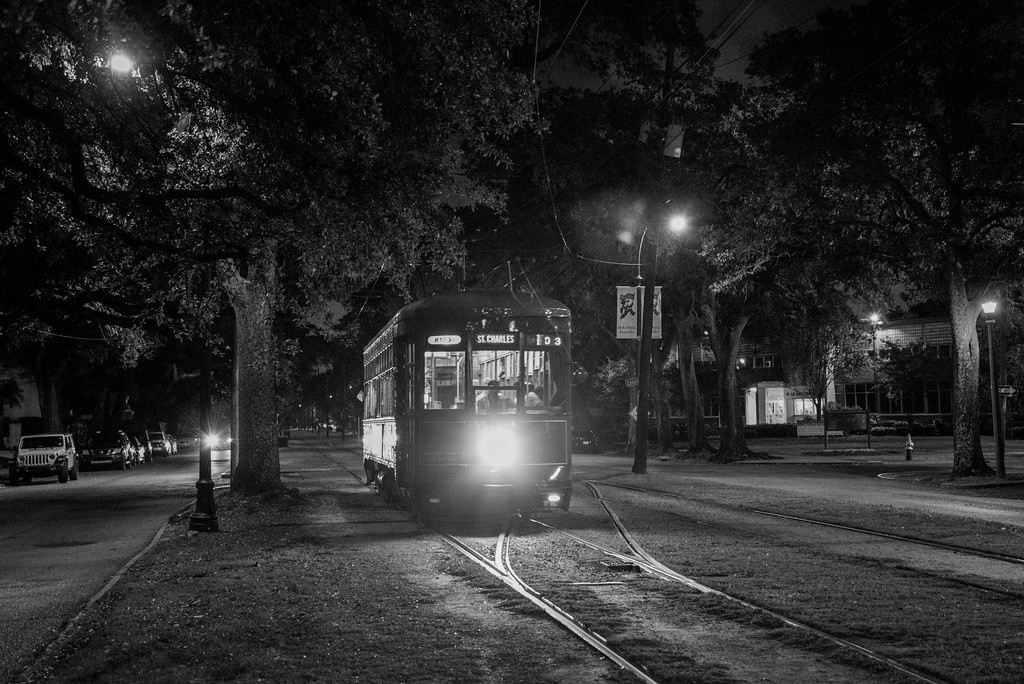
[870,314,882,413]
[632,200,689,476]
[981,297,1007,480]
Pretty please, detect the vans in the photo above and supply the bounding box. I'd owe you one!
[128,432,153,461]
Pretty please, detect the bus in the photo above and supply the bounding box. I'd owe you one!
[361,285,571,513]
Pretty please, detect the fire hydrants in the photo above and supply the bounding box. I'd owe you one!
[905,435,914,462]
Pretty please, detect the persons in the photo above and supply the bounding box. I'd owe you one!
[525,386,543,406]
[475,377,502,411]
[476,380,516,413]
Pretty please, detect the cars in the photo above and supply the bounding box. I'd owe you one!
[127,436,145,465]
[572,429,598,452]
[212,430,231,451]
[178,433,200,448]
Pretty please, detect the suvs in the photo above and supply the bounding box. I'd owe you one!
[146,431,178,456]
[82,430,131,470]
[11,434,80,484]
